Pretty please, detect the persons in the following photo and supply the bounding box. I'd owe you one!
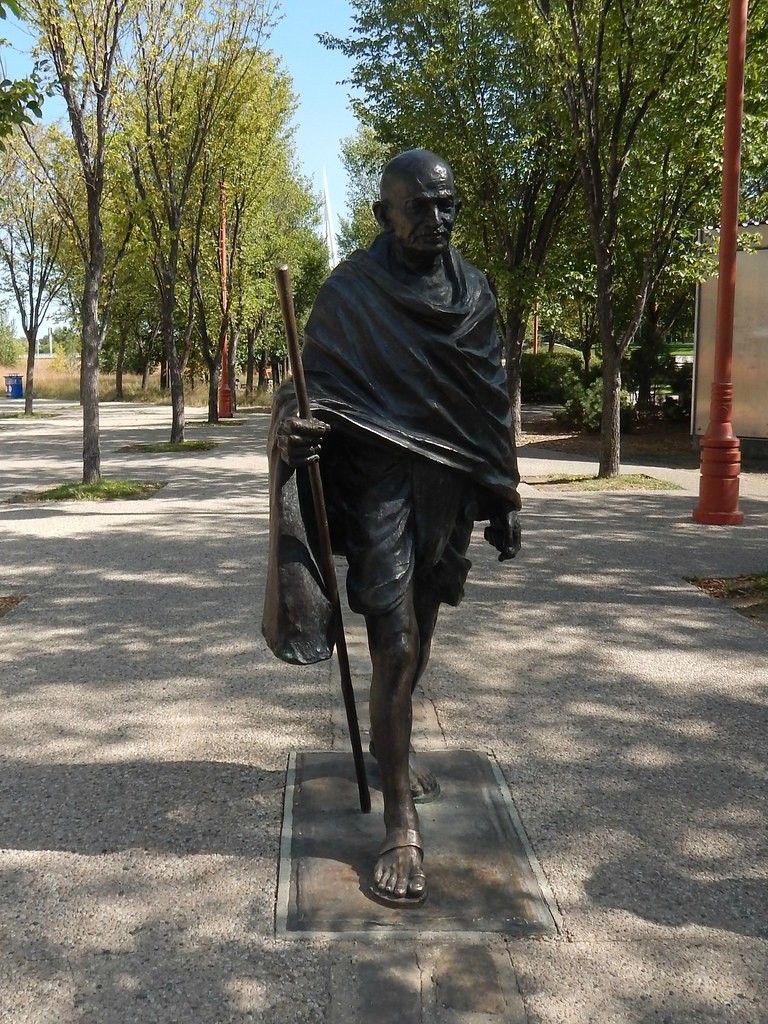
[266,149,521,910]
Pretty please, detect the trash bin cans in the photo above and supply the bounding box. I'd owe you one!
[3,372,23,399]
